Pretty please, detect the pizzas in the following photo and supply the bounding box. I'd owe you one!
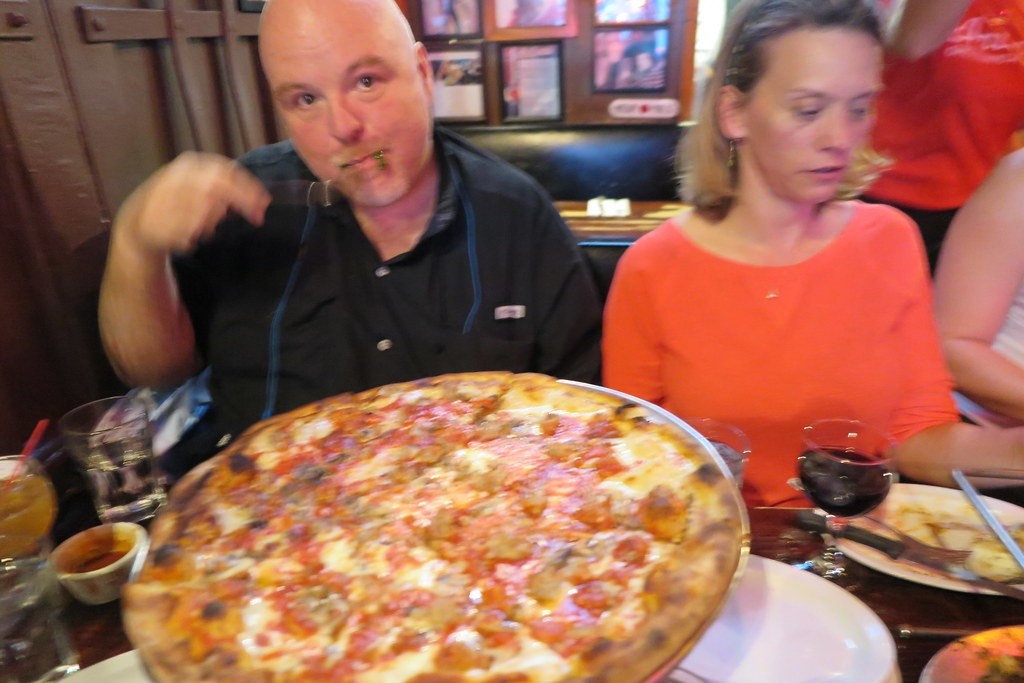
[120,371,741,683]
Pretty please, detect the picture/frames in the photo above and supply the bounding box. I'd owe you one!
[590,0,677,28]
[418,0,484,42]
[496,38,565,125]
[482,0,579,43]
[589,24,673,95]
[425,43,489,127]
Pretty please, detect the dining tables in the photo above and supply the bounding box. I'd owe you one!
[0,488,1024,683]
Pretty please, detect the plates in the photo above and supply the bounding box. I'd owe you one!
[914,625,1024,683]
[811,485,1024,594]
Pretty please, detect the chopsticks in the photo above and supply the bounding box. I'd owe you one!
[952,469,1024,566]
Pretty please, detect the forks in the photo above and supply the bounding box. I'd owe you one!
[866,514,971,564]
[788,478,971,566]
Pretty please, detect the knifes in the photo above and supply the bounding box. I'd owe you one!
[790,511,1024,600]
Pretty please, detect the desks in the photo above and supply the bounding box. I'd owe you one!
[553,200,696,249]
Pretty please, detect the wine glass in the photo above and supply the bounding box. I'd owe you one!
[799,417,898,596]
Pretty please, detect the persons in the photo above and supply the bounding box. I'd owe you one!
[602,1,1024,505]
[99,0,597,484]
[850,1,1024,423]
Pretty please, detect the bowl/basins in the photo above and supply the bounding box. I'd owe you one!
[51,521,144,606]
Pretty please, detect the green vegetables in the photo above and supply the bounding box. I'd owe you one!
[337,153,389,175]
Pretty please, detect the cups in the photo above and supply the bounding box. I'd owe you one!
[56,396,169,524]
[0,556,79,683]
[0,454,55,559]
[681,416,750,490]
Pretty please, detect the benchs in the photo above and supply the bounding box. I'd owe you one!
[457,122,682,297]
